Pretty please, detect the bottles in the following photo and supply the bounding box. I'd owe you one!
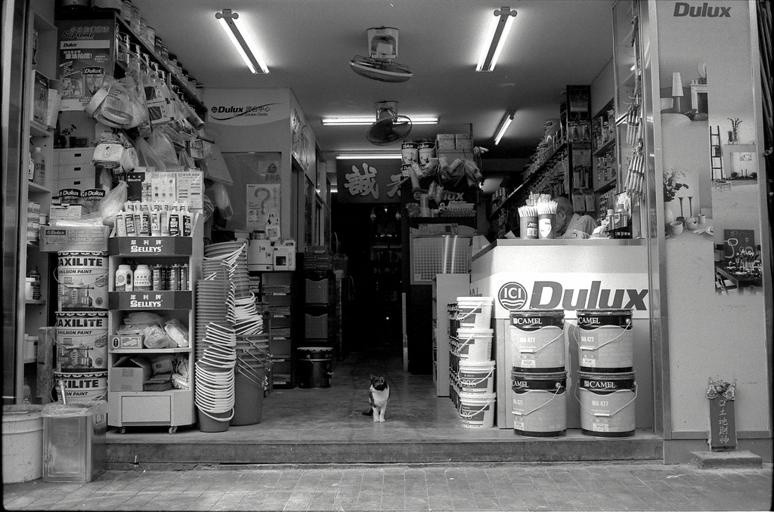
[31,146,47,186]
[26,264,42,301]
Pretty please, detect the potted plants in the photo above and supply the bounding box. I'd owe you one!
[727,116,743,144]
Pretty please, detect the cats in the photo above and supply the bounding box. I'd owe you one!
[361,374,391,424]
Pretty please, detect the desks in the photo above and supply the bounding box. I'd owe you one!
[715,261,761,293]
[722,144,755,178]
[689,83,707,114]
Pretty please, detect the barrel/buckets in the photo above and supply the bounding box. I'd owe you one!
[400,141,437,178]
[446,296,496,428]
[574,308,639,437]
[297,346,335,388]
[1,396,46,484]
[51,249,109,433]
[195,239,276,431]
[508,306,569,437]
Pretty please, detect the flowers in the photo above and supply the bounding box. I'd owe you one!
[663,168,689,201]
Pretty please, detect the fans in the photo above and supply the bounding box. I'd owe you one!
[369,100,412,146]
[347,27,413,86]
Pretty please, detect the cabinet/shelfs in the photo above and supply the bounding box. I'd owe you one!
[54,3,209,131]
[586,96,617,193]
[5,67,46,404]
[103,206,204,433]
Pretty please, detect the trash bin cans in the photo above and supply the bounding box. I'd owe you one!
[2,403,107,485]
[299,347,332,387]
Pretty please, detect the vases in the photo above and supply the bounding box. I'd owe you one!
[665,202,674,232]
[669,214,706,236]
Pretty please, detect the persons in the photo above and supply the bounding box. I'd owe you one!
[548,196,598,239]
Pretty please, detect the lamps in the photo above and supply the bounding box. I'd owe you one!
[493,111,517,145]
[476,7,517,72]
[321,117,440,126]
[214,8,271,75]
[671,71,684,113]
